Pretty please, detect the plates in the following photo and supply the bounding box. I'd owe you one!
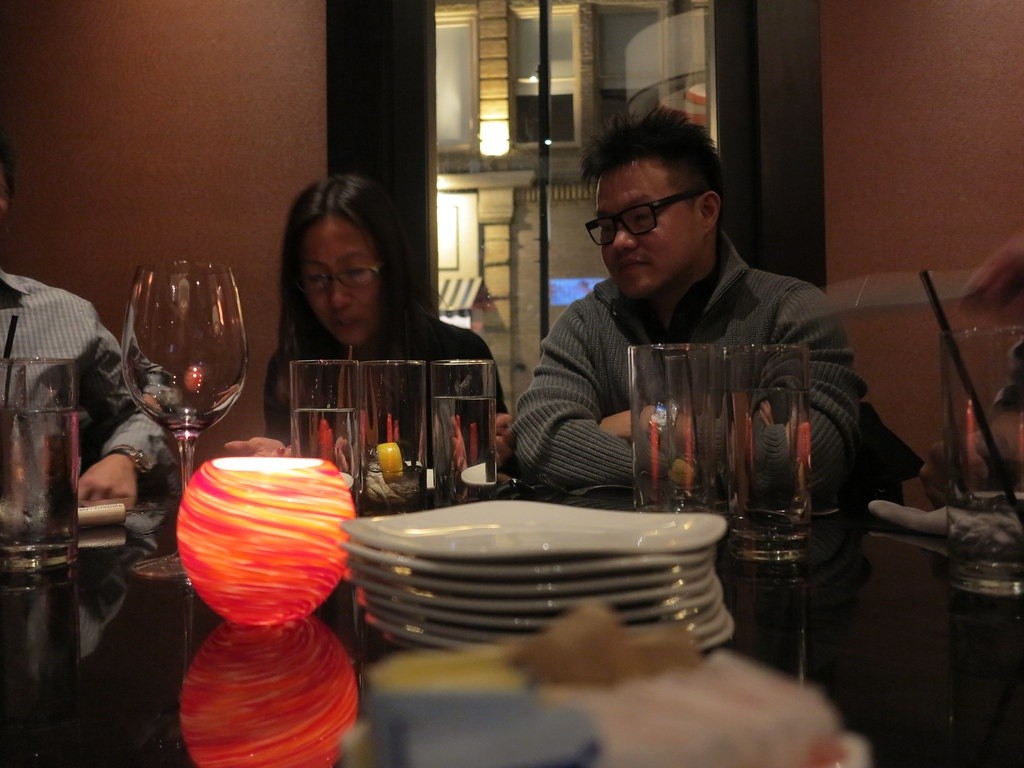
[342,645,871,768]
[340,500,734,650]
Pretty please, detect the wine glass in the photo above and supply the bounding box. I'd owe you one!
[121,260,248,578]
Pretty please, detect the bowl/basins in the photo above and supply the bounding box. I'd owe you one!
[460,463,514,486]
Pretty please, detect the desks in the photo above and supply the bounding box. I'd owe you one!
[0,491,1023,766]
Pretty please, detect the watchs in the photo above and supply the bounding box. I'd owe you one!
[104,448,152,474]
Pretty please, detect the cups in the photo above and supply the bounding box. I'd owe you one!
[429,360,496,508]
[938,326,1024,595]
[665,340,731,501]
[289,360,359,516]
[723,344,814,560]
[0,356,83,574]
[627,344,709,513]
[359,360,425,504]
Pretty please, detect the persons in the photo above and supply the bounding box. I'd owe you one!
[1,131,185,510]
[512,108,867,500]
[223,176,518,473]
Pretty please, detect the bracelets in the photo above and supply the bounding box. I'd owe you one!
[649,400,679,435]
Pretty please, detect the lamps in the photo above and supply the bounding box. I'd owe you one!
[177,457,355,626]
[181,615,355,767]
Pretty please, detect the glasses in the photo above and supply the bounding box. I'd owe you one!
[585,186,705,245]
[292,255,387,293]
